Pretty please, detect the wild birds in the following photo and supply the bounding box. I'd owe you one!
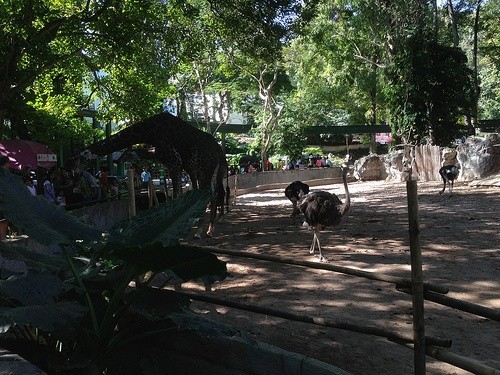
[296,167,354,263]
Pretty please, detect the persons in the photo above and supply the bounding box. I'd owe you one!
[0,154,364,242]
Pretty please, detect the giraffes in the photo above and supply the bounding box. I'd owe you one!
[73,112,230,239]
